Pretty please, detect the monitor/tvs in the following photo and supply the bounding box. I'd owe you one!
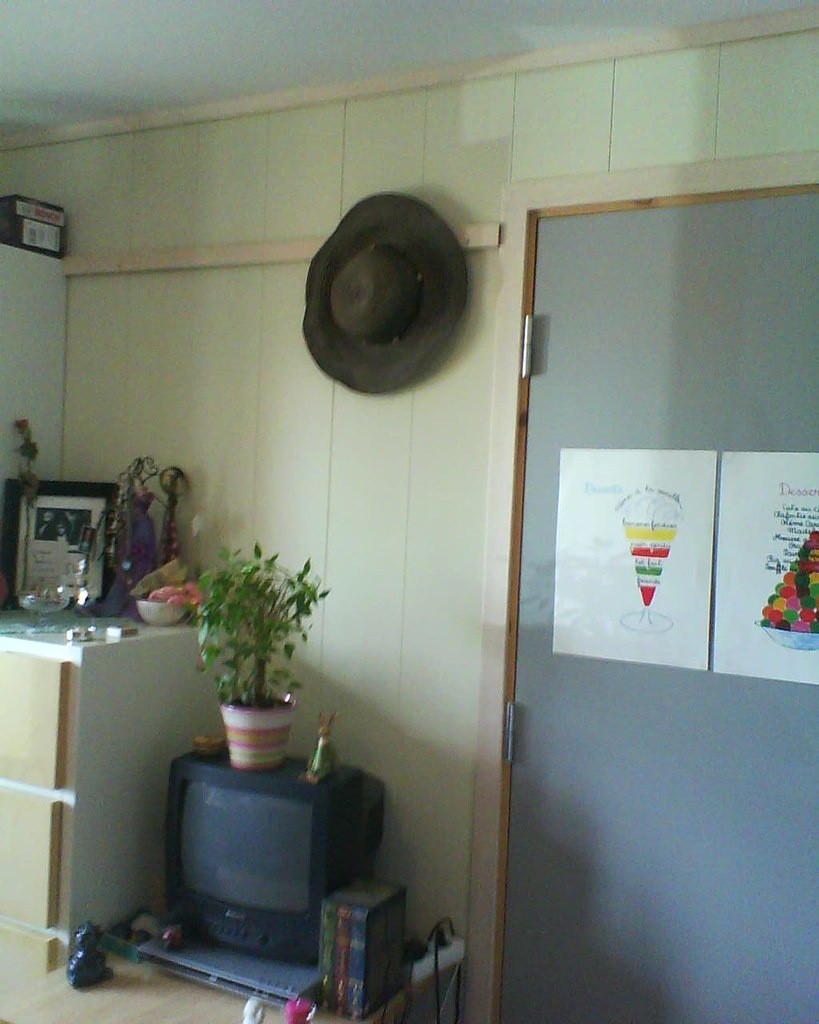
[165,749,386,968]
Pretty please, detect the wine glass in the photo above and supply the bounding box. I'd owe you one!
[18,578,71,632]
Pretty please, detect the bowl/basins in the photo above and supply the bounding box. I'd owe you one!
[134,598,188,625]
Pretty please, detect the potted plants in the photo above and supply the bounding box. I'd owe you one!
[191,542,329,771]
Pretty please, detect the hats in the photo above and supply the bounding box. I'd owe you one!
[303,193,468,394]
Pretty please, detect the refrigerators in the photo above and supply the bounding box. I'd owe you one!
[0,610,234,1006]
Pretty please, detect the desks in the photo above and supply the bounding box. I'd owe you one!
[0,601,256,972]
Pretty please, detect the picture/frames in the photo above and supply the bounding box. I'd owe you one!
[0,478,120,608]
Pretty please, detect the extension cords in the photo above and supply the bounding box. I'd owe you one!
[401,935,466,984]
